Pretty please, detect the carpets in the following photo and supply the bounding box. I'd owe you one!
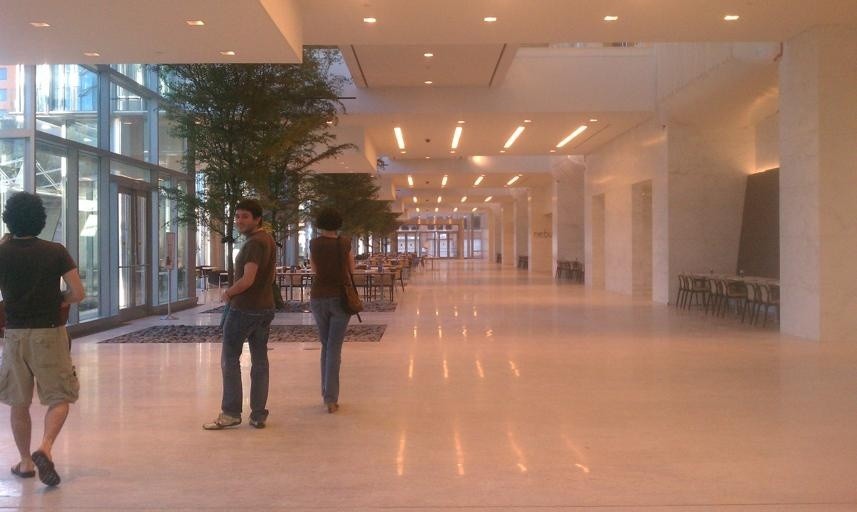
[97,323,388,343]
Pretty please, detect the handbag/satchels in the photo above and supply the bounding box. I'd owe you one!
[219,281,284,330]
[342,284,363,314]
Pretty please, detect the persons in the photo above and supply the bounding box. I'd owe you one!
[0,190,86,487]
[307,208,356,414]
[202,199,278,429]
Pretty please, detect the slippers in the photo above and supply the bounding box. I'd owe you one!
[32,451,60,486]
[11,462,35,477]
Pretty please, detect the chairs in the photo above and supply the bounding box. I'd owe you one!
[200,251,428,323]
[555,259,584,286]
[675,272,779,328]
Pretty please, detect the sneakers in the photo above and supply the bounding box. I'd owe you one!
[203,413,241,429]
[328,404,339,412]
[248,416,265,428]
